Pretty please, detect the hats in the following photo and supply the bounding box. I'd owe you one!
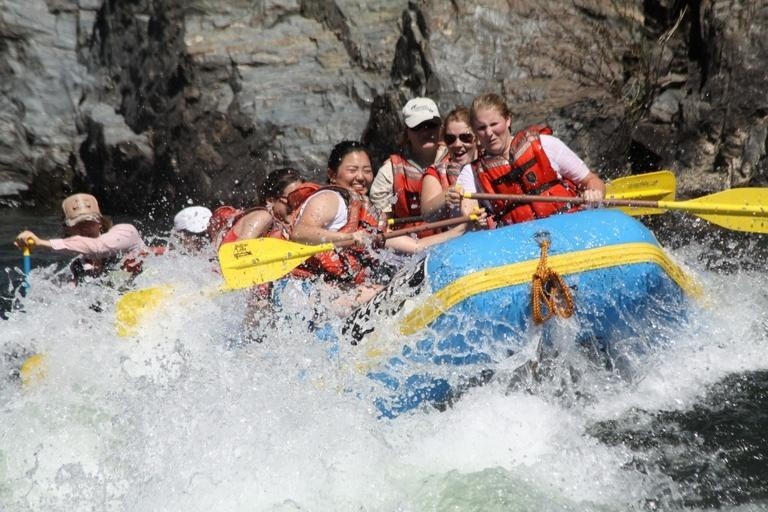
[173,206,212,234]
[402,96,440,128]
[60,193,103,228]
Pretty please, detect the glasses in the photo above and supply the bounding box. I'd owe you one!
[443,133,472,145]
[411,121,438,132]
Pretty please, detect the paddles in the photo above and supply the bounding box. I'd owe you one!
[218,214,479,291]
[604,170,676,218]
[115,282,177,338]
[14,239,48,391]
[454,187,768,235]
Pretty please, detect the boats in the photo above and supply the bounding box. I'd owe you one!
[16,284,175,386]
[290,200,711,428]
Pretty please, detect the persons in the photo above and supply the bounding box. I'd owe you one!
[270,138,489,325]
[119,205,218,273]
[12,193,155,313]
[363,96,446,240]
[449,93,607,230]
[209,166,310,256]
[417,103,478,222]
